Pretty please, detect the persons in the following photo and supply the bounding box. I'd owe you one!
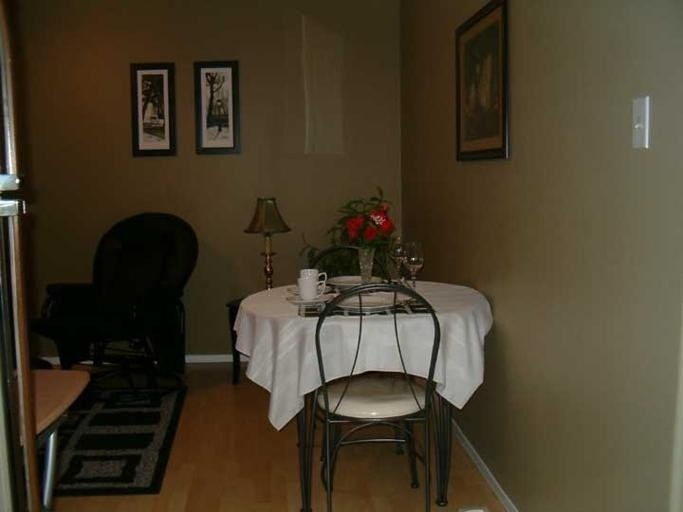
[210,99,225,134]
[143,90,161,120]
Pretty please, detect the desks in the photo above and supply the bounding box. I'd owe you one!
[13,367,91,511]
[224,296,245,386]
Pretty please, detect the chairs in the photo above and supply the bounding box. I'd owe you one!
[306,245,416,463]
[40,211,199,368]
[303,283,448,512]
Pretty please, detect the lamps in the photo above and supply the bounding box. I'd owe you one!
[244,196,292,290]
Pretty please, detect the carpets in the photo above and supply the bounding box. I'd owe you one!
[35,383,187,496]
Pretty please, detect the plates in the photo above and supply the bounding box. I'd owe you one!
[288,296,334,304]
[337,296,402,312]
[327,275,383,288]
[287,286,333,293]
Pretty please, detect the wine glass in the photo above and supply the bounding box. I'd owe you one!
[390,237,424,290]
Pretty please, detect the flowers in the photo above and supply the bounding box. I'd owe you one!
[324,184,395,242]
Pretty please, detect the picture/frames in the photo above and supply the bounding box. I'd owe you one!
[453,0,510,161]
[193,60,240,155]
[130,62,177,156]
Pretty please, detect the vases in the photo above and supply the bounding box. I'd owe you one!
[358,248,376,284]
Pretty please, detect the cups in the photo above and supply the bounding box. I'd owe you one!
[297,269,327,300]
